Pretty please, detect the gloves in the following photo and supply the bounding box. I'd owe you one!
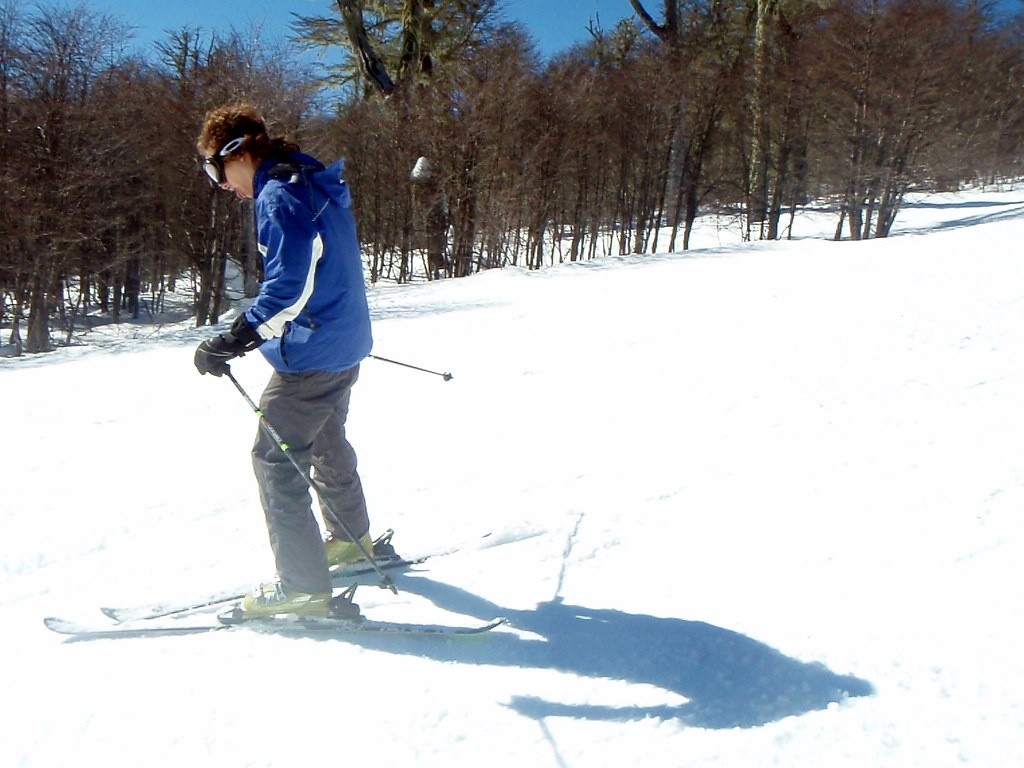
[194,312,267,376]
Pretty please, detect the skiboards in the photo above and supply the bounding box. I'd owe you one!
[98,523,549,626]
[41,615,508,636]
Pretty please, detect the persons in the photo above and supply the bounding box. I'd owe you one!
[194,104,374,618]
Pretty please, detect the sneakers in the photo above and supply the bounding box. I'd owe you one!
[324,530,373,563]
[244,588,332,615]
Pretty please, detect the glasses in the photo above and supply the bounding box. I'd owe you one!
[203,134,252,183]
